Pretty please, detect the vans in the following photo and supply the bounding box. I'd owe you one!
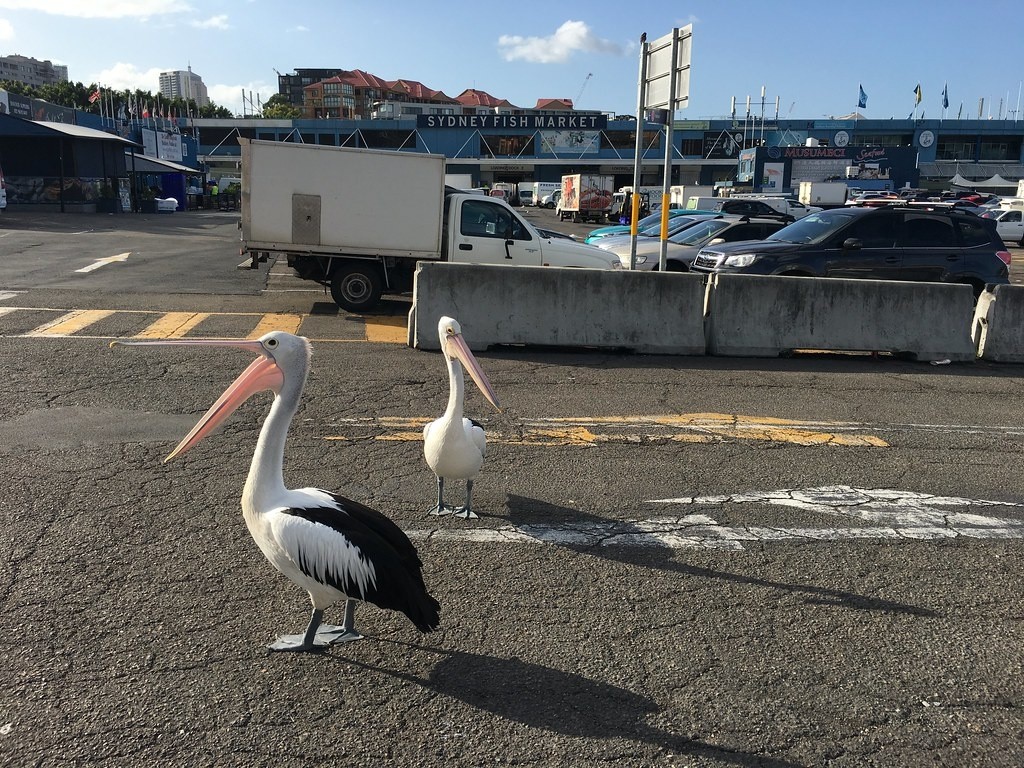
[218,178,240,196]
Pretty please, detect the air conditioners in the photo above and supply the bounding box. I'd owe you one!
[465,155,474,158]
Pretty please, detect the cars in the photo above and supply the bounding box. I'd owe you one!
[582,184,1024,271]
[0,165,8,212]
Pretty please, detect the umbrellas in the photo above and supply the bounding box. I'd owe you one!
[948,173,1018,187]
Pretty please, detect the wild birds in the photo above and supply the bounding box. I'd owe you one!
[423,314,500,521]
[109,331,443,652]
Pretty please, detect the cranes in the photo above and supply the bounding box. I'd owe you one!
[571,72,593,110]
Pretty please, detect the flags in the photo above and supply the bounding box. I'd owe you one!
[913,81,921,105]
[942,80,949,109]
[88,88,178,126]
[858,83,868,108]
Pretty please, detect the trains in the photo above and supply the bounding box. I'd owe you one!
[370,101,602,122]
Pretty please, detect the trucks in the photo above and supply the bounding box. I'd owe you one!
[619,184,713,210]
[445,173,517,206]
[555,174,615,223]
[517,182,535,207]
[532,183,561,208]
[541,189,561,208]
[234,134,627,315]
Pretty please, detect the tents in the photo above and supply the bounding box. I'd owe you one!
[0,112,146,215]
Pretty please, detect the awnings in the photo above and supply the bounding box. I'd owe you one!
[125,151,200,172]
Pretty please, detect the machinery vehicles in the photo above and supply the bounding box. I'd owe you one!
[613,193,653,225]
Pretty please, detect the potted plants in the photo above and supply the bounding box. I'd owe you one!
[99,185,122,213]
[141,186,159,214]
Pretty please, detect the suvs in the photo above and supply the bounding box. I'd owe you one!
[686,201,1012,318]
